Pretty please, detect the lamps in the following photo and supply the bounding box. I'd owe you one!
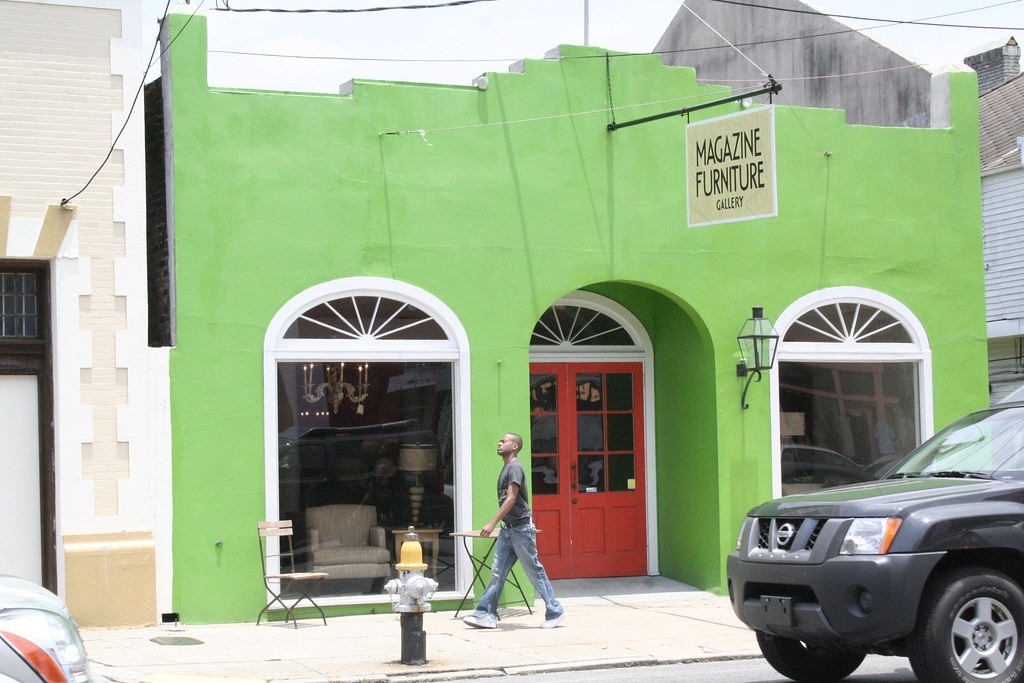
[737,306,780,410]
[397,440,439,527]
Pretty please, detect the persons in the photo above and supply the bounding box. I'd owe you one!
[463,433,567,629]
[348,439,415,595]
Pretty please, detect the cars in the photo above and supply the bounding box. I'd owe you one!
[781,444,880,496]
[0,575,90,683]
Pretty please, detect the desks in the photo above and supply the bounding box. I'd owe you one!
[392,525,444,583]
[449,526,543,619]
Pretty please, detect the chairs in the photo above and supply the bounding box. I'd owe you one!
[303,504,389,592]
[255,520,327,629]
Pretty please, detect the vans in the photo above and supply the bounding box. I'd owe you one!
[279,418,446,567]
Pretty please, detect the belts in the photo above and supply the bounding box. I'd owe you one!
[505,517,532,529]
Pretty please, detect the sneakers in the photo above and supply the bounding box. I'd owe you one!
[463,616,496,629]
[541,609,567,629]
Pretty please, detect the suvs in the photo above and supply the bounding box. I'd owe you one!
[726,401,1024,683]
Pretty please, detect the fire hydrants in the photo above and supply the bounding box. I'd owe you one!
[383,526,439,666]
